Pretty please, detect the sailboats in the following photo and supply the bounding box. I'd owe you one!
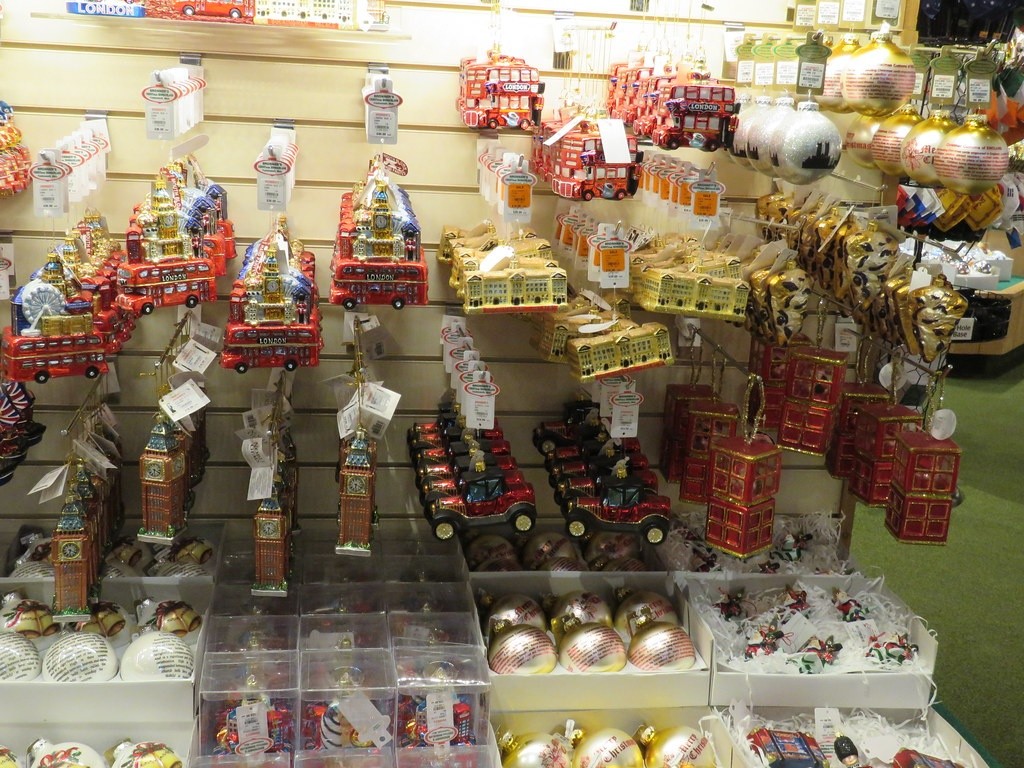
[0,377,47,486]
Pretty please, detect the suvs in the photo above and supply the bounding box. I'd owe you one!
[406,400,672,544]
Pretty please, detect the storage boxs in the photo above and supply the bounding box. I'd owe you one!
[2,510,1008,768]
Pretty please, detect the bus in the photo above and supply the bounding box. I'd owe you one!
[329,152,428,310]
[457,41,741,201]
[218,213,324,374]
[1,152,237,386]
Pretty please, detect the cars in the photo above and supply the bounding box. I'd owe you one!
[174,0,246,18]
